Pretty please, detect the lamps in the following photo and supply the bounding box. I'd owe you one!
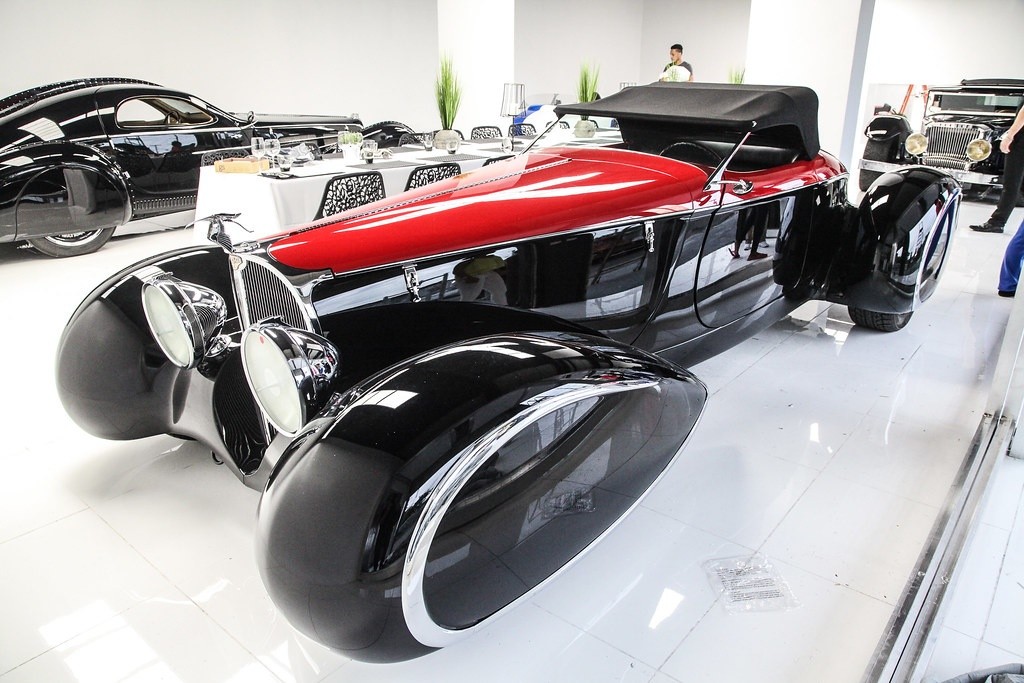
[499,83,526,152]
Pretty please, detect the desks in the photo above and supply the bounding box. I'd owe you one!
[192,127,627,239]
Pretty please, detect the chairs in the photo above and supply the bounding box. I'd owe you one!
[201,119,620,221]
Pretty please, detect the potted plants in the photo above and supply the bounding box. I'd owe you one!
[575,58,603,138]
[434,51,464,150]
[339,130,363,166]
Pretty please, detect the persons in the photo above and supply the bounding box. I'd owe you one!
[999,221,1024,298]
[663,44,693,82]
[451,249,508,306]
[728,204,769,261]
[968,105,1024,233]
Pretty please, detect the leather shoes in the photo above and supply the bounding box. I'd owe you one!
[969,221,1004,233]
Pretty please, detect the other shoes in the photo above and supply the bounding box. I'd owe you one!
[998,291,1016,297]
[744,244,751,250]
[728,248,740,258]
[747,252,768,261]
[759,241,769,248]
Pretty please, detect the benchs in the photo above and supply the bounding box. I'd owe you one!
[961,79,1024,87]
[625,135,802,172]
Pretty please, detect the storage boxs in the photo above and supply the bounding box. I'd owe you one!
[214,158,269,173]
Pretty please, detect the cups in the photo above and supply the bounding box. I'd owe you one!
[362,140,375,164]
[367,142,378,152]
[424,140,432,150]
[277,155,293,172]
[447,140,457,153]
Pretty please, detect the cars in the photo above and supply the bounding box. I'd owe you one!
[0,76,360,258]
[856,79,1024,204]
[47,77,965,668]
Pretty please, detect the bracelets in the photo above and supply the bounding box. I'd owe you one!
[1005,133,1012,140]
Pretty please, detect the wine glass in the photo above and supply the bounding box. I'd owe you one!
[251,137,265,174]
[265,139,280,170]
